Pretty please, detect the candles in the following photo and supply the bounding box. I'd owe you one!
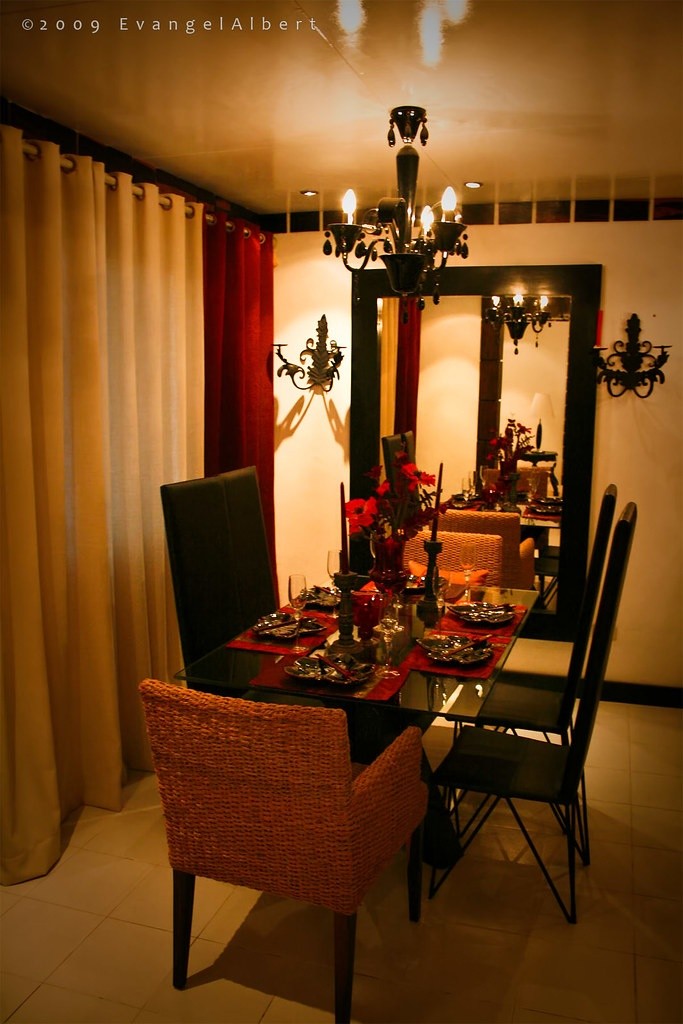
[337,482,349,570]
[428,465,445,540]
[596,310,604,347]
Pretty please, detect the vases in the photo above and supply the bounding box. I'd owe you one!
[500,459,518,479]
[366,533,411,584]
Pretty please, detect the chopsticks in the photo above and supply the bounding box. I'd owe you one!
[314,585,344,599]
[465,604,517,615]
[315,654,359,682]
[440,634,493,658]
[253,617,314,632]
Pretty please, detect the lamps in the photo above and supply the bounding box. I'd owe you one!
[327,106,470,325]
[481,291,562,358]
[523,391,555,452]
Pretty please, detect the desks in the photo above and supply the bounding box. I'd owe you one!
[435,495,561,606]
[514,452,559,496]
[170,581,541,926]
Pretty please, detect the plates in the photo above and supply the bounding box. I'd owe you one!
[448,604,514,623]
[532,497,562,515]
[451,493,476,509]
[284,652,377,686]
[300,587,341,607]
[252,613,327,639]
[405,577,446,595]
[416,635,493,664]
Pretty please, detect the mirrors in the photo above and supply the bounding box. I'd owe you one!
[349,255,605,624]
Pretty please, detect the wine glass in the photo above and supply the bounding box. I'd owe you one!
[462,465,489,510]
[460,541,476,604]
[327,550,342,618]
[527,471,540,509]
[289,575,309,653]
[433,565,451,635]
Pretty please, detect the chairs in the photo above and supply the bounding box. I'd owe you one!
[532,544,561,613]
[380,432,422,519]
[140,677,431,1024]
[451,481,618,835]
[406,531,499,585]
[422,502,639,925]
[484,467,550,500]
[161,464,389,736]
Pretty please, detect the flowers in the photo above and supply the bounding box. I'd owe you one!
[486,425,533,460]
[366,449,418,537]
[345,464,435,544]
